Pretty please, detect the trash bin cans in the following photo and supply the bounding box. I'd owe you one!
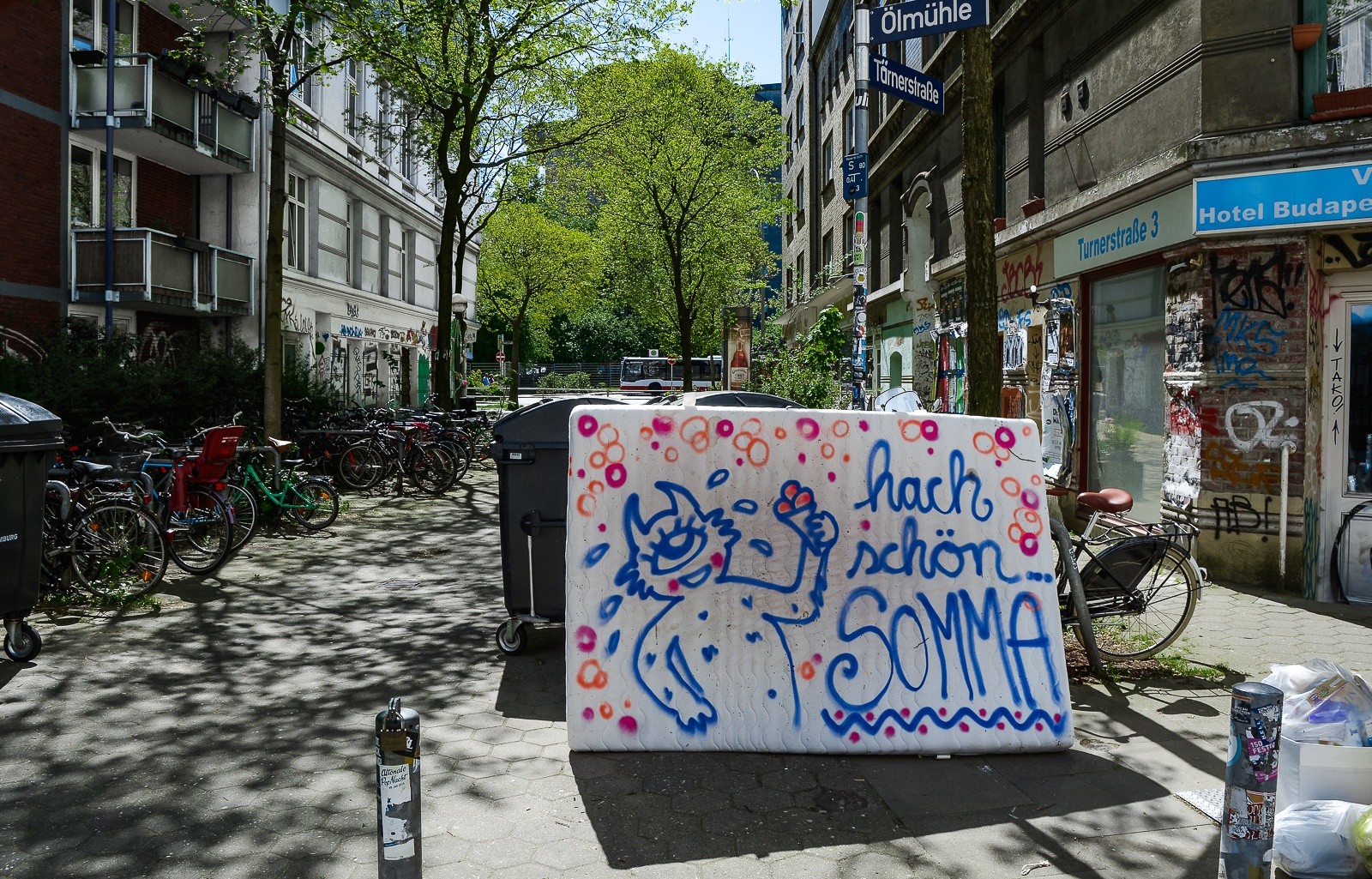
[0,390,63,661]
[490,398,629,657]
[644,390,808,408]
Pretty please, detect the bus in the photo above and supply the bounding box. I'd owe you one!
[619,354,724,396]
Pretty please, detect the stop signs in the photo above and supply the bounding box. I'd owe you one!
[667,354,677,365]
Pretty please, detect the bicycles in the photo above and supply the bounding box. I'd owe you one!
[38,378,498,603]
[1046,485,1213,664]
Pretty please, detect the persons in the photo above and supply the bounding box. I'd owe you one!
[483,373,494,385]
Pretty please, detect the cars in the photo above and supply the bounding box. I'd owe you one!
[646,390,807,409]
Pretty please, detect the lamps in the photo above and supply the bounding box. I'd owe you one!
[1030,285,1050,309]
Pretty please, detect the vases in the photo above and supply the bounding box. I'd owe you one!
[175,236,209,252]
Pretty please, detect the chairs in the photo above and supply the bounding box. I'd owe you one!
[169,424,247,513]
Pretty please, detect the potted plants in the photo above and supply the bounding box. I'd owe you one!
[156,47,262,119]
[69,48,105,65]
